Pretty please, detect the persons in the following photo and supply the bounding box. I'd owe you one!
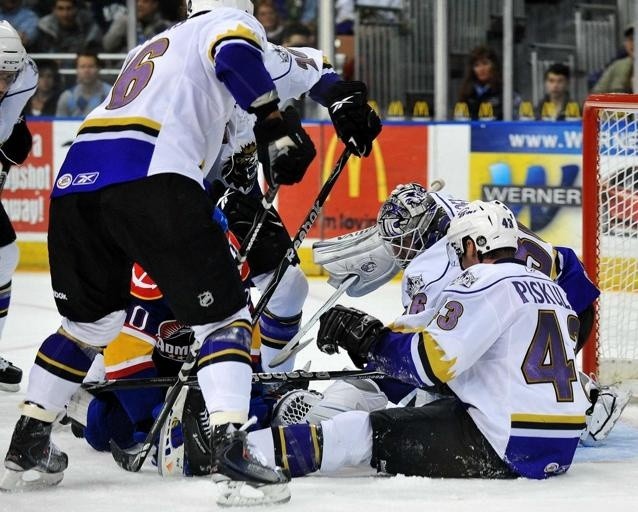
[0,21,42,394]
[157,200,587,482]
[297,182,633,448]
[534,63,579,118]
[33,1,105,55]
[61,206,268,453]
[53,50,116,118]
[104,0,175,56]
[0,1,39,53]
[22,61,63,118]
[250,0,287,46]
[453,46,504,123]
[204,39,383,374]
[278,21,312,49]
[0,0,313,511]
[591,23,635,97]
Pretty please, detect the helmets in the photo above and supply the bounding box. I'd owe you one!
[183,0,256,19]
[0,19,27,94]
[377,181,443,270]
[446,199,519,267]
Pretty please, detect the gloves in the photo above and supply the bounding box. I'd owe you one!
[325,81,384,159]
[317,303,385,367]
[254,108,317,187]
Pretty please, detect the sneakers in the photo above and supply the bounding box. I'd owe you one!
[209,424,293,486]
[182,390,213,478]
[589,380,633,443]
[3,401,69,472]
[0,356,23,385]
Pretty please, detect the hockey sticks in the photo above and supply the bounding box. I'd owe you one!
[264,176,448,367]
[106,149,295,472]
[62,367,389,429]
